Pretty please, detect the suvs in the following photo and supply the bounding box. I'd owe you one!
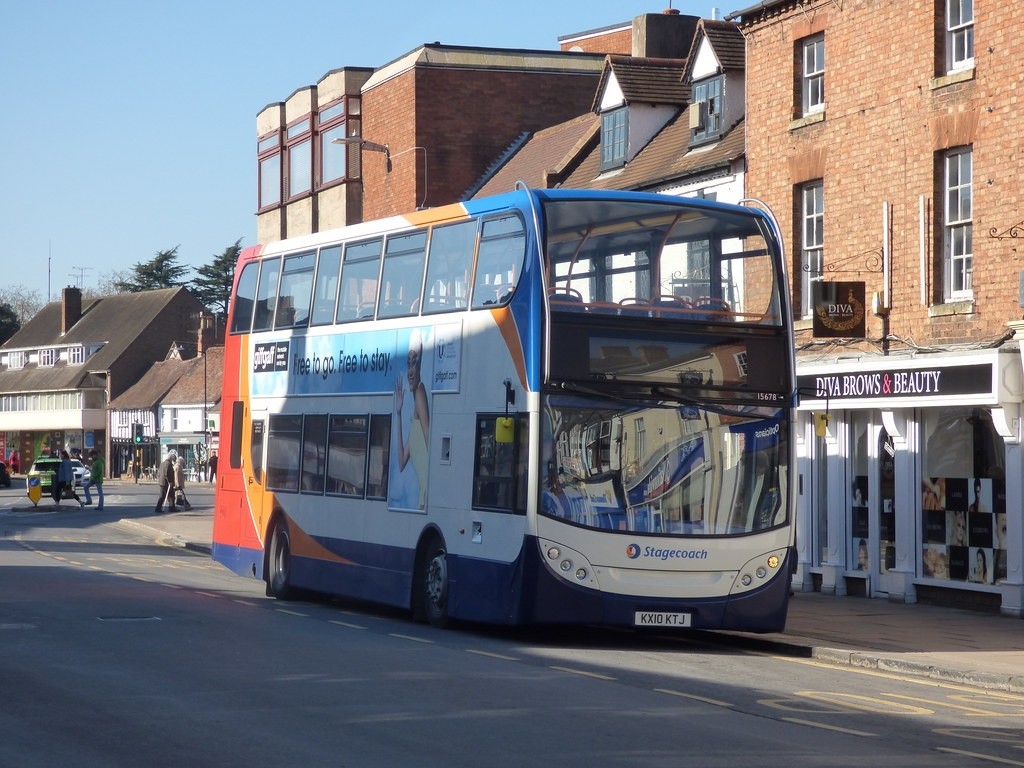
[26,456,75,499]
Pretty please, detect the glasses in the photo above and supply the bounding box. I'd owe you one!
[407,357,419,368]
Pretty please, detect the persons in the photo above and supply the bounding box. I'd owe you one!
[84,449,105,511]
[391,334,431,512]
[851,482,867,506]
[923,545,948,578]
[8,449,19,476]
[950,511,966,547]
[207,451,218,484]
[922,479,946,511]
[853,540,867,569]
[993,513,1007,550]
[53,449,85,510]
[968,478,987,513]
[154,449,181,513]
[971,547,994,583]
[167,456,195,512]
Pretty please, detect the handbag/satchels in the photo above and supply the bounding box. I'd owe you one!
[175,489,186,505]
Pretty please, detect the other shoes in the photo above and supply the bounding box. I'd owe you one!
[53,504,61,510]
[81,502,85,510]
[95,506,104,510]
[86,500,92,505]
[184,508,194,511]
[155,509,164,513]
[169,509,180,512]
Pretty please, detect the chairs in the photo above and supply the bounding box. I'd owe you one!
[268,288,732,328]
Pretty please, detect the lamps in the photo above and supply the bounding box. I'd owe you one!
[330,136,389,154]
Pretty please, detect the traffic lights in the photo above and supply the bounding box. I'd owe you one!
[131,423,144,444]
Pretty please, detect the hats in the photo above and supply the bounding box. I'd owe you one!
[409,328,422,360]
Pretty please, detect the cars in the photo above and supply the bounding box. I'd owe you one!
[69,459,91,487]
[0,462,11,487]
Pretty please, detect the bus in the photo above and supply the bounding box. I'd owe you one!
[212,179,833,634]
